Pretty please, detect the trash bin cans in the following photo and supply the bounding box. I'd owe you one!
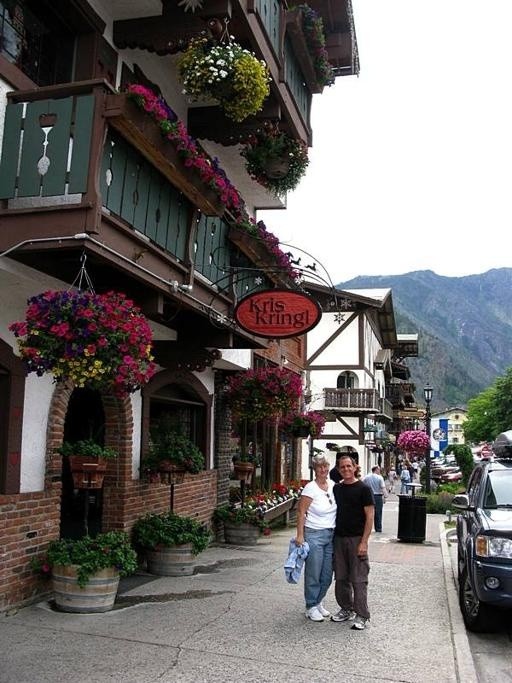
[396,483,427,543]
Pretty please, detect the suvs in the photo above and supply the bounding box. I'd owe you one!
[451,429,512,633]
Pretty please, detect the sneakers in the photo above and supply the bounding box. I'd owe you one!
[331,608,357,621]
[305,602,330,621]
[351,615,367,629]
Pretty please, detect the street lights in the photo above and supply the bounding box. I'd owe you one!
[423,383,433,495]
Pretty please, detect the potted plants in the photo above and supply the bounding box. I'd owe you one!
[52,438,121,489]
[214,504,270,547]
[233,454,264,484]
[138,430,206,485]
[132,509,214,577]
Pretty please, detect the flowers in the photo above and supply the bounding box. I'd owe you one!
[285,2,335,92]
[396,430,431,457]
[7,291,159,400]
[29,527,139,590]
[125,82,299,279]
[223,364,327,440]
[244,479,310,512]
[173,20,272,123]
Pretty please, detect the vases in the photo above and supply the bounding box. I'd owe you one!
[50,563,120,614]
[208,68,239,102]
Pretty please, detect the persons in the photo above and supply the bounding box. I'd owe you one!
[296,451,331,622]
[361,465,389,533]
[389,459,427,497]
[331,455,375,630]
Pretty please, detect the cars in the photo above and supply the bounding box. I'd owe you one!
[434,441,493,487]
